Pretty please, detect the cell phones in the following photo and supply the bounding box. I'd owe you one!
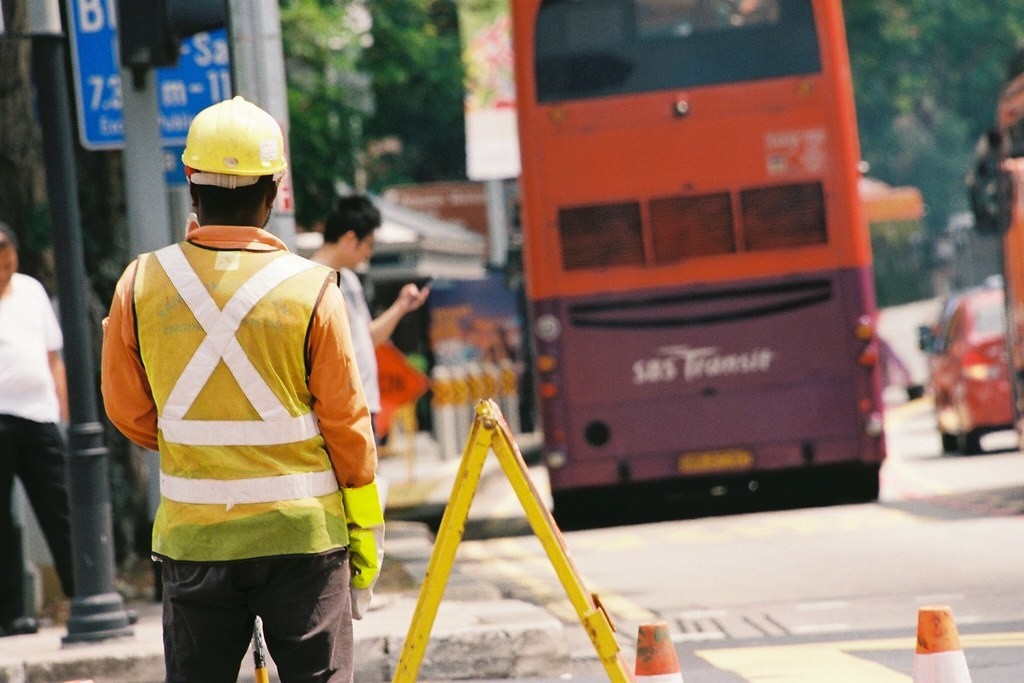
[417,275,436,291]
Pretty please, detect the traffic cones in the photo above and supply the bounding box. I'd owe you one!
[908,606,974,683]
[633,624,682,683]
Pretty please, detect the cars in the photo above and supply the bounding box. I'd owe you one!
[918,288,1012,454]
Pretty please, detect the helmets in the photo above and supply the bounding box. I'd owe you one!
[180,95,288,177]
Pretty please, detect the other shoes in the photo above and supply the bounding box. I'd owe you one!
[1,617,37,636]
[104,605,139,626]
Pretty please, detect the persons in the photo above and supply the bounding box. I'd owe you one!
[0,222,139,638]
[96,94,387,683]
[310,195,431,455]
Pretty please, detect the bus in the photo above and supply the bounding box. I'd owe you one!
[506,1,887,527]
